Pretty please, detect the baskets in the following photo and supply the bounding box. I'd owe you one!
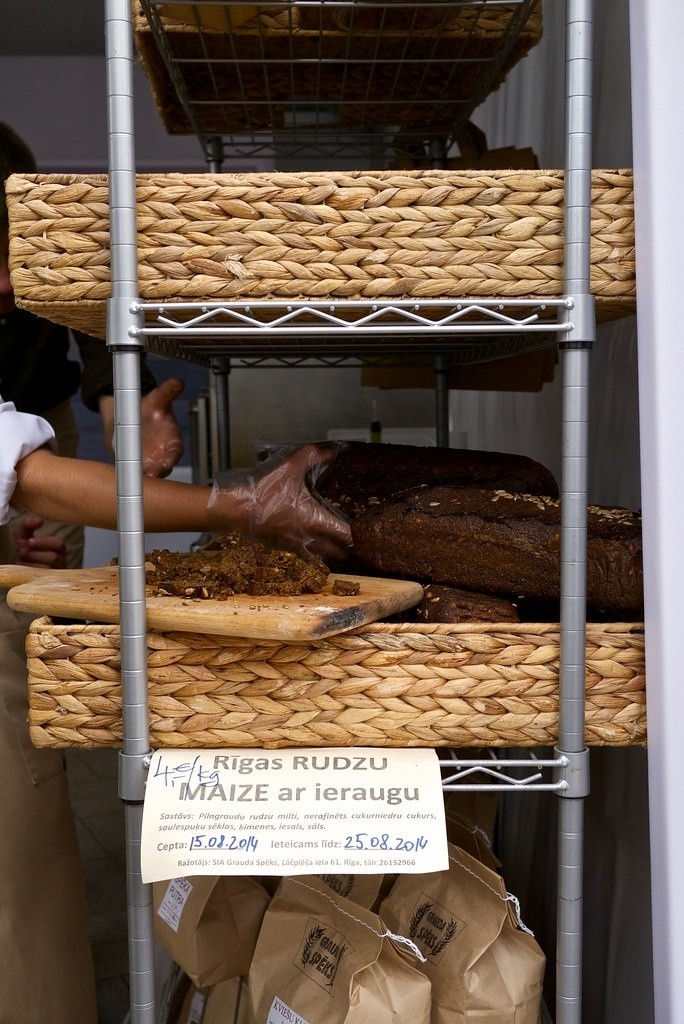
[4,169,566,342]
[23,615,648,750]
[131,0,546,137]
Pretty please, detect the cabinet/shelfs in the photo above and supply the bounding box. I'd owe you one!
[106,0,596,1024]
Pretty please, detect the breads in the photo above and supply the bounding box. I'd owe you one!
[146,439,644,625]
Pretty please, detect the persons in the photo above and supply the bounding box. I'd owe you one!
[0,121,185,638]
[0,389,354,1024]
